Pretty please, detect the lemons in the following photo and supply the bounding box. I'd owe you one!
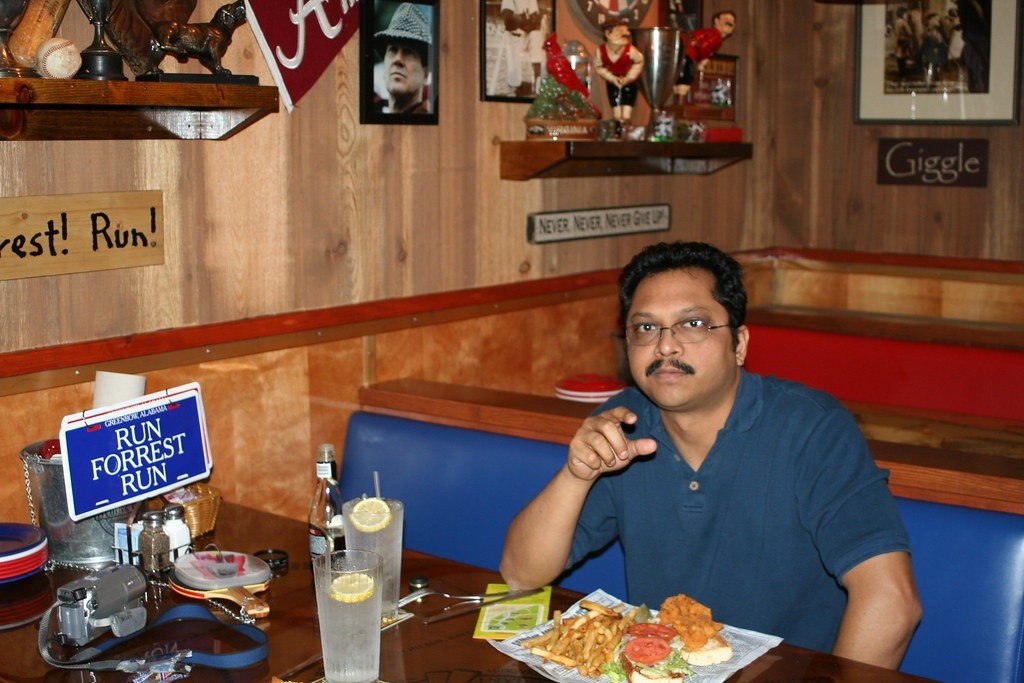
[350,497,392,532]
[330,573,375,602]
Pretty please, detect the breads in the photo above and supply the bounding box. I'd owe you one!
[679,631,733,665]
[620,652,684,683]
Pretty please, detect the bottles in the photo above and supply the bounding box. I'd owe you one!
[562,39,592,98]
[308,444,345,624]
[163,503,191,563]
[139,511,169,572]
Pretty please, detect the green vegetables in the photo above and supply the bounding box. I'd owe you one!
[598,615,695,683]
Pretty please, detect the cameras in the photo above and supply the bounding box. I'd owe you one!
[56,563,150,647]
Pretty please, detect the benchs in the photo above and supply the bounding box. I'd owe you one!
[745,303,1024,418]
[327,376,1024,682]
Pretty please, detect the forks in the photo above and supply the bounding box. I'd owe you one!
[398,588,523,607]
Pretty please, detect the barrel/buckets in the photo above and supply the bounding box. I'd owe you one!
[19,440,142,570]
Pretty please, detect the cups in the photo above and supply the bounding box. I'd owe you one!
[313,549,383,683]
[342,497,404,623]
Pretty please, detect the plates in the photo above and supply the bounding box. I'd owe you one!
[0,522,48,584]
[524,663,561,683]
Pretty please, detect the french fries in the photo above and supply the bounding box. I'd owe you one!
[521,600,637,678]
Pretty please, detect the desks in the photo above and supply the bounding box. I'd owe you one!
[851,404,1023,480]
[0,501,936,683]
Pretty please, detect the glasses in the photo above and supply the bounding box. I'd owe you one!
[625,320,737,346]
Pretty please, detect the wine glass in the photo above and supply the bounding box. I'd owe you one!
[628,27,682,141]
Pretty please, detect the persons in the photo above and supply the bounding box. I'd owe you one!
[593,19,644,130]
[673,11,736,95]
[374,3,432,114]
[499,241,922,670]
[896,0,991,93]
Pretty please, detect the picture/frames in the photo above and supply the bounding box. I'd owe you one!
[479,0,556,102]
[358,0,439,125]
[854,0,1024,125]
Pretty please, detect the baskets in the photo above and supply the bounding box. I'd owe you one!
[164,484,222,541]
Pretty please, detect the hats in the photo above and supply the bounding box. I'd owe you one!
[372,2,432,46]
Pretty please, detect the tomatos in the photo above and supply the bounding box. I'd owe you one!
[625,635,672,664]
[628,623,674,642]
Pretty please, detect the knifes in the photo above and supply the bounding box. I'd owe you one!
[423,587,545,625]
